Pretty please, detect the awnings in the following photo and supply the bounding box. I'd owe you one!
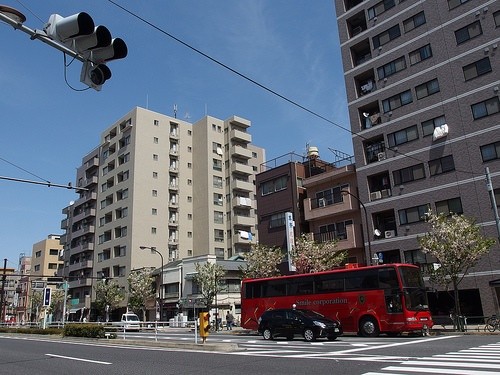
[65,308,81,313]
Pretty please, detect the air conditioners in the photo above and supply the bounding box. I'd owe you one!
[75,190,89,257]
[370,191,382,201]
[384,230,395,239]
[381,189,391,198]
[378,152,386,161]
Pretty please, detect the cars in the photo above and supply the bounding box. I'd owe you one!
[258,308,343,342]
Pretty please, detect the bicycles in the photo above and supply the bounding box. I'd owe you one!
[460,314,468,333]
[484,315,500,333]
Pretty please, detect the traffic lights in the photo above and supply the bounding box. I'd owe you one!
[43,287,51,307]
[46,11,125,92]
[199,312,212,337]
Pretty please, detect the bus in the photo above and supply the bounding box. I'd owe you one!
[240,264,434,337]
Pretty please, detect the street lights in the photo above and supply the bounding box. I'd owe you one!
[140,246,163,326]
[340,189,374,266]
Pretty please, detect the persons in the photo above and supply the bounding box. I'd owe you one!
[226,311,234,330]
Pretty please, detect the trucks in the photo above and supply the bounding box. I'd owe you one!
[121,313,141,330]
